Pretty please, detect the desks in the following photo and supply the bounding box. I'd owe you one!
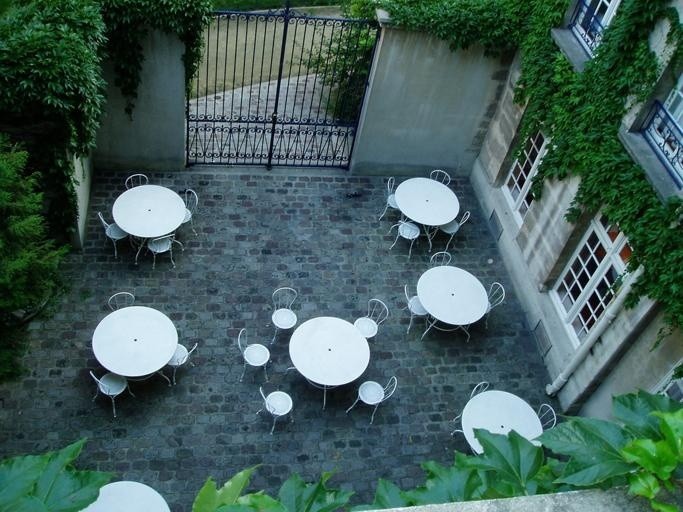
[283,316,371,411]
[91,306,179,387]
[416,265,488,343]
[461,390,544,454]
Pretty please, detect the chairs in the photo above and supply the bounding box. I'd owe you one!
[168,342,198,386]
[108,292,135,312]
[485,282,505,329]
[353,299,389,339]
[256,386,294,436]
[238,328,270,383]
[430,252,452,268]
[470,382,489,400]
[379,169,470,259]
[90,370,136,418]
[345,376,398,425]
[537,404,557,431]
[266,287,298,345]
[402,284,429,334]
[98,174,199,269]
